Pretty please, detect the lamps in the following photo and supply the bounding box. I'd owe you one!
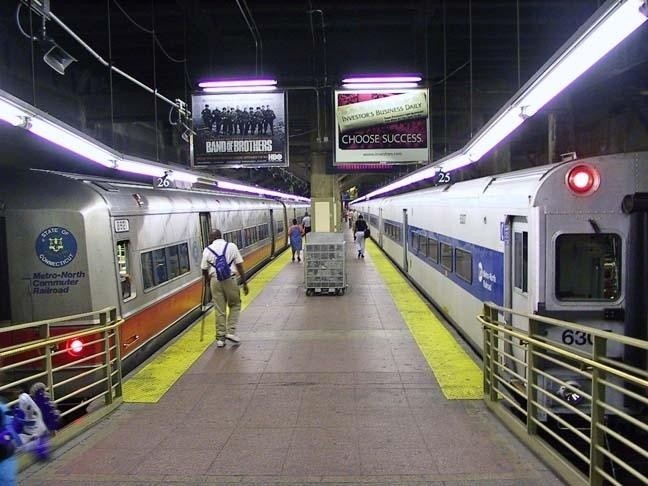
[341,1,647,207]
[0,20,312,206]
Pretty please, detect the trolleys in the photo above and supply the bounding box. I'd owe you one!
[303,232,348,296]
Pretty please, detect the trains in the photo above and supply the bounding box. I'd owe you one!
[348,151,648,431]
[0,167,311,406]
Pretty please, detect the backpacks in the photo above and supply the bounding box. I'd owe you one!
[206,243,235,280]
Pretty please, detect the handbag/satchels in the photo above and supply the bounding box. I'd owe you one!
[364,229,370,238]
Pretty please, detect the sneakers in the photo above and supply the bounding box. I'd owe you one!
[216,341,225,347]
[225,334,241,342]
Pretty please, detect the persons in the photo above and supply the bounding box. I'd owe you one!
[287,219,305,261]
[344,208,358,228]
[302,212,311,234]
[355,215,368,258]
[201,228,250,347]
[201,103,278,139]
